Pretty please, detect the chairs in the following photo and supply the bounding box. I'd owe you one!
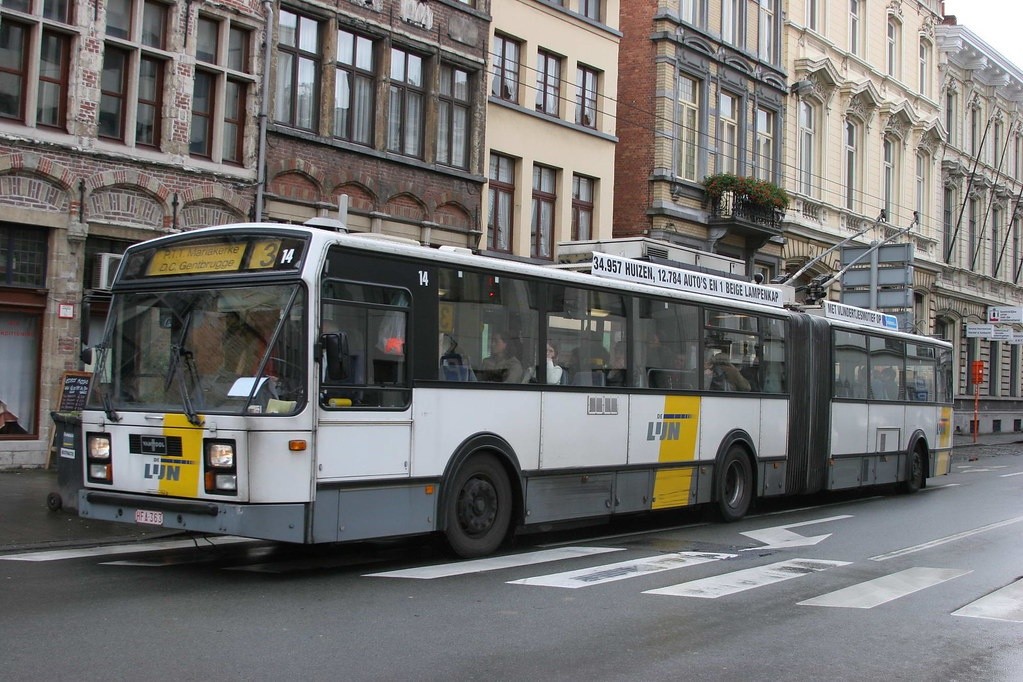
[339,339,941,399]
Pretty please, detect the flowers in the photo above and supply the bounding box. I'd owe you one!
[702,172,789,209]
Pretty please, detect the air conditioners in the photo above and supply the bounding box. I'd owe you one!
[91,252,124,290]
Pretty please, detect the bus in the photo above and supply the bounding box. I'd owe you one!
[75,217,954,560]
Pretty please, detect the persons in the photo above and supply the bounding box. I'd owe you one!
[481,330,523,383]
[569,327,899,403]
[523,340,564,383]
[218,309,376,389]
